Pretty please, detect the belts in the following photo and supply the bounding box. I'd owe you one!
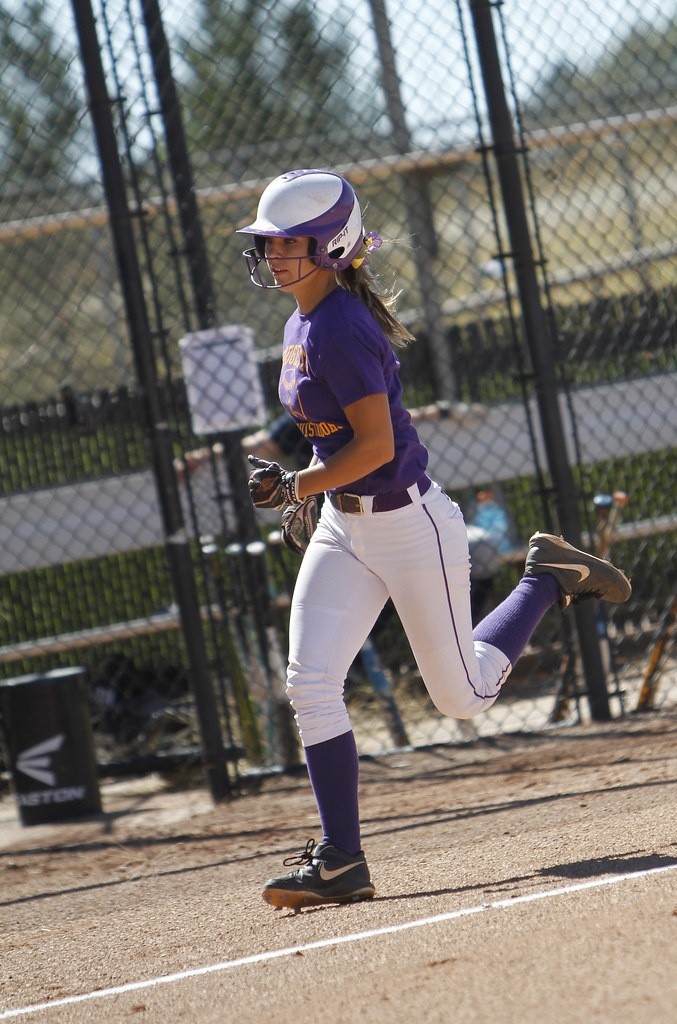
[326,474,431,514]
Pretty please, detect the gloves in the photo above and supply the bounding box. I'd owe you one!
[247,455,307,511]
[280,493,326,555]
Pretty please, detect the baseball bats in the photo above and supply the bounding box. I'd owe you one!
[637,592,677,712]
[549,490,629,725]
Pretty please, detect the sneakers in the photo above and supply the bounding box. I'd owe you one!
[523,531,632,612]
[261,838,376,909]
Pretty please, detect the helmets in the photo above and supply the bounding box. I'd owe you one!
[235,168,364,288]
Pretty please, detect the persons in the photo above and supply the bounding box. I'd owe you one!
[547,492,611,724]
[472,483,512,556]
[235,170,631,907]
